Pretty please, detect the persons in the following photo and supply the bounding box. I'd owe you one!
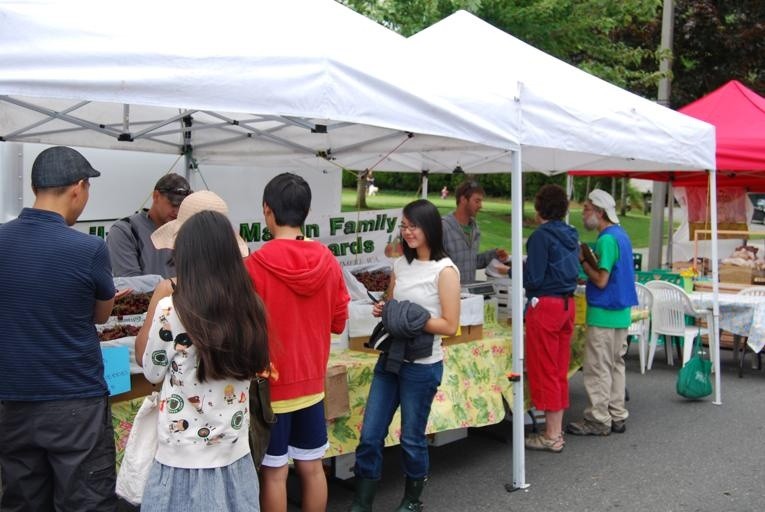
[136,191,269,511]
[443,179,509,292]
[102,171,192,294]
[576,190,640,436]
[2,145,119,511]
[526,183,578,453]
[354,197,461,511]
[243,172,350,509]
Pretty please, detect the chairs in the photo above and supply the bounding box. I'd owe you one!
[623,277,764,379]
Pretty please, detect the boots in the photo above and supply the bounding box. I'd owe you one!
[344,474,382,511]
[395,474,431,512]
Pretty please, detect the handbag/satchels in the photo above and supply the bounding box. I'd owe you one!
[246,376,275,473]
[677,334,712,399]
[116,392,163,508]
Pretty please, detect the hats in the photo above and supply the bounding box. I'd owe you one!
[150,191,248,259]
[31,147,101,188]
[155,174,194,206]
[588,189,620,223]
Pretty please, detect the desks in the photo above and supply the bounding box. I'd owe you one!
[98,285,650,512]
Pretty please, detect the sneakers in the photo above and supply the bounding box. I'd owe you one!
[567,419,611,436]
[612,420,625,432]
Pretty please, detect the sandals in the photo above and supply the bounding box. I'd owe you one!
[524,432,568,452]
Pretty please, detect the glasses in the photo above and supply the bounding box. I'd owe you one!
[398,224,417,231]
[469,181,482,187]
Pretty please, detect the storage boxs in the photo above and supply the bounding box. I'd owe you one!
[345,291,391,356]
[98,326,154,404]
[441,293,486,349]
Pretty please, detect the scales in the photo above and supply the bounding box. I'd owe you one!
[461,281,496,295]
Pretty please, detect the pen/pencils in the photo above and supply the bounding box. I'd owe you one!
[367,291,379,303]
